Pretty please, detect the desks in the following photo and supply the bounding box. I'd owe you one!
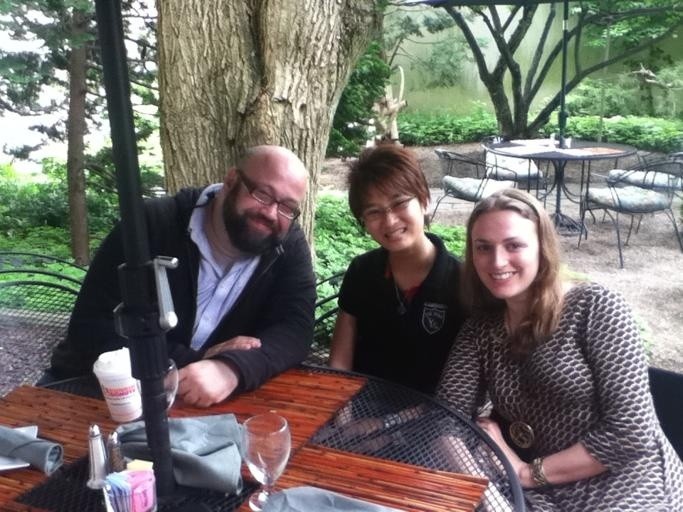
[492,140,634,234]
[0,362,526,512]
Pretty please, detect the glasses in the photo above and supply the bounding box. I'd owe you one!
[360,195,419,224]
[236,169,301,221]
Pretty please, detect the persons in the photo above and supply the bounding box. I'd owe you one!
[311,146,466,468]
[427,186,683,512]
[35,144,317,411]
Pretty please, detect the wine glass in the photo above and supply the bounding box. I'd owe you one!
[244,416,290,511]
[136,358,178,421]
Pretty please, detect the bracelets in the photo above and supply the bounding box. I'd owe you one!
[530,456,552,490]
[383,414,404,430]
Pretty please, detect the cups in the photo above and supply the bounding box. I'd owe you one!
[93,357,143,425]
[102,472,156,511]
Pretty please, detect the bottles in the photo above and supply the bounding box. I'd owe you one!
[82,423,125,488]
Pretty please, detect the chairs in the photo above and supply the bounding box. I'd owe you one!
[428,149,517,223]
[1,251,89,394]
[481,135,548,206]
[309,267,349,362]
[575,161,681,269]
[604,153,682,232]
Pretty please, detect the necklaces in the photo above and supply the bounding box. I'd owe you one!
[392,264,409,320]
